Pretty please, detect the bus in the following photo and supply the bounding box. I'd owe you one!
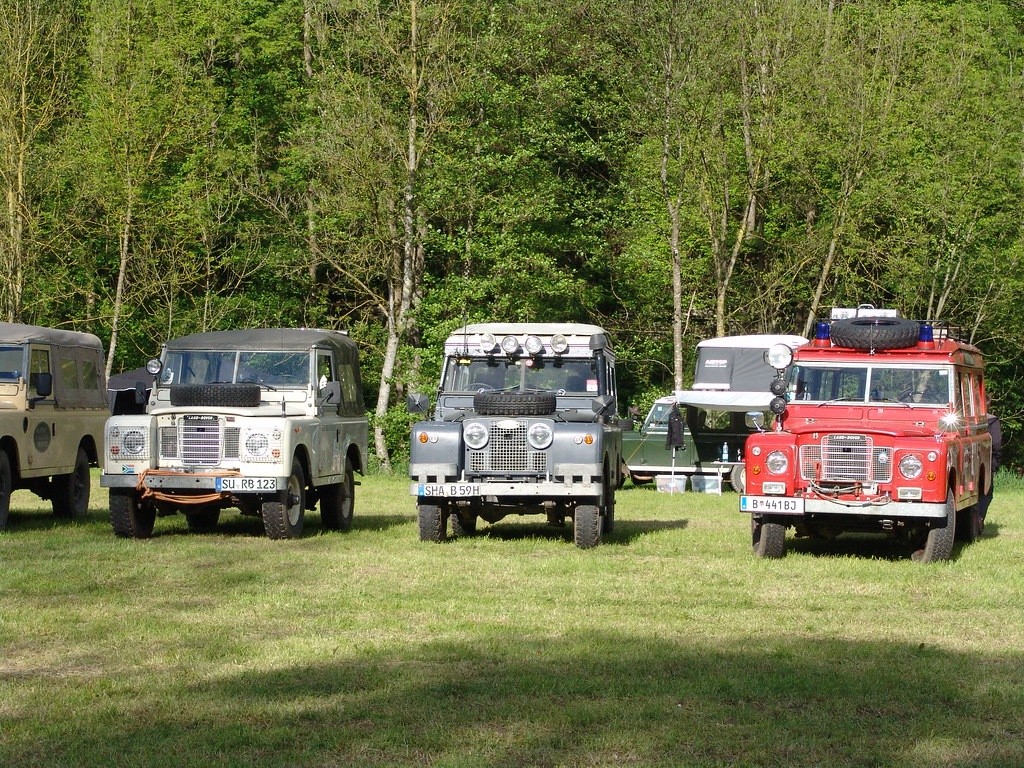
[692,335,811,393]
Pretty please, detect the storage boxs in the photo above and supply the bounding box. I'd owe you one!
[655,474,687,493]
[689,474,723,493]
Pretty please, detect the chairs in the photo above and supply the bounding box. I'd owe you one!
[564,376,583,392]
[472,373,495,392]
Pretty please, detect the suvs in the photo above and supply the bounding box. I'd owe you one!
[99,328,368,540]
[405,322,623,551]
[617,396,773,493]
[0,322,111,532]
[739,316,993,565]
